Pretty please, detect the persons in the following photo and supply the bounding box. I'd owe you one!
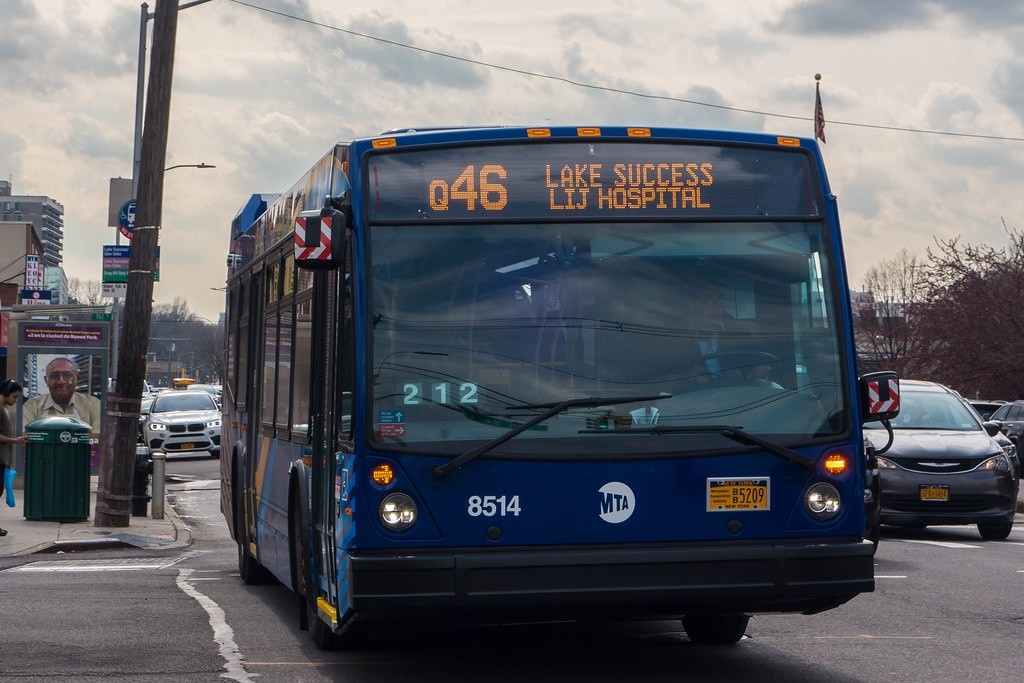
[662,286,772,382]
[0,379,28,536]
[22,357,101,439]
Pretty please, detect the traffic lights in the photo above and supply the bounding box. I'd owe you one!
[214,370,217,374]
[206,372,209,379]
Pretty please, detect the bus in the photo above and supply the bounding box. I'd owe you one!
[217,124,900,658]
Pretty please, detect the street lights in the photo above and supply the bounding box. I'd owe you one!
[177,351,194,378]
[112,163,220,389]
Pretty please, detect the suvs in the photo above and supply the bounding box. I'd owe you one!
[965,399,1009,425]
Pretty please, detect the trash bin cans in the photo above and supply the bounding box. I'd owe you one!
[23,416,92,521]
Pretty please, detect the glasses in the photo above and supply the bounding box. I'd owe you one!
[48,373,75,380]
[696,295,726,307]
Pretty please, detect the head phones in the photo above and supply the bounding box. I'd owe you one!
[3,380,15,397]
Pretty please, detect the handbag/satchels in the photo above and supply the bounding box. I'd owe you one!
[5,467,17,507]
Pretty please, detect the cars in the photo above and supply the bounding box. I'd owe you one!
[139,388,221,460]
[864,378,1019,539]
[988,400,1024,477]
[137,370,225,442]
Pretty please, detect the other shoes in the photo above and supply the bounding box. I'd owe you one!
[0,528,8,536]
